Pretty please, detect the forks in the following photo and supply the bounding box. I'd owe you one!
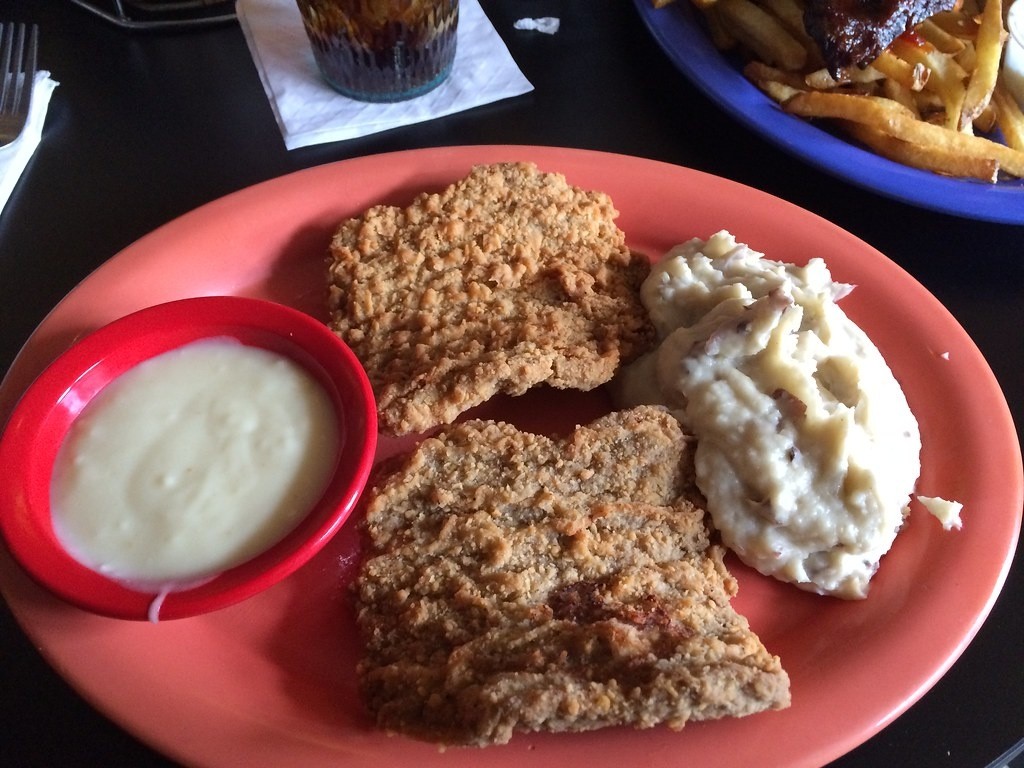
[0,22,39,147]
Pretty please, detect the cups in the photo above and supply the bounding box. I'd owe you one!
[296,0,460,104]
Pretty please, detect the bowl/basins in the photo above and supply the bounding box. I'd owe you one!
[0,294,378,622]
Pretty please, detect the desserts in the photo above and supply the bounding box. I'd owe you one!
[619,232,921,605]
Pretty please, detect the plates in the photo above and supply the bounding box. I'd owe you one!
[0,146,1024,768]
[634,0,1024,228]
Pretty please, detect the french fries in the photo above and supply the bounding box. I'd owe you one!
[656,0,1024,183]
